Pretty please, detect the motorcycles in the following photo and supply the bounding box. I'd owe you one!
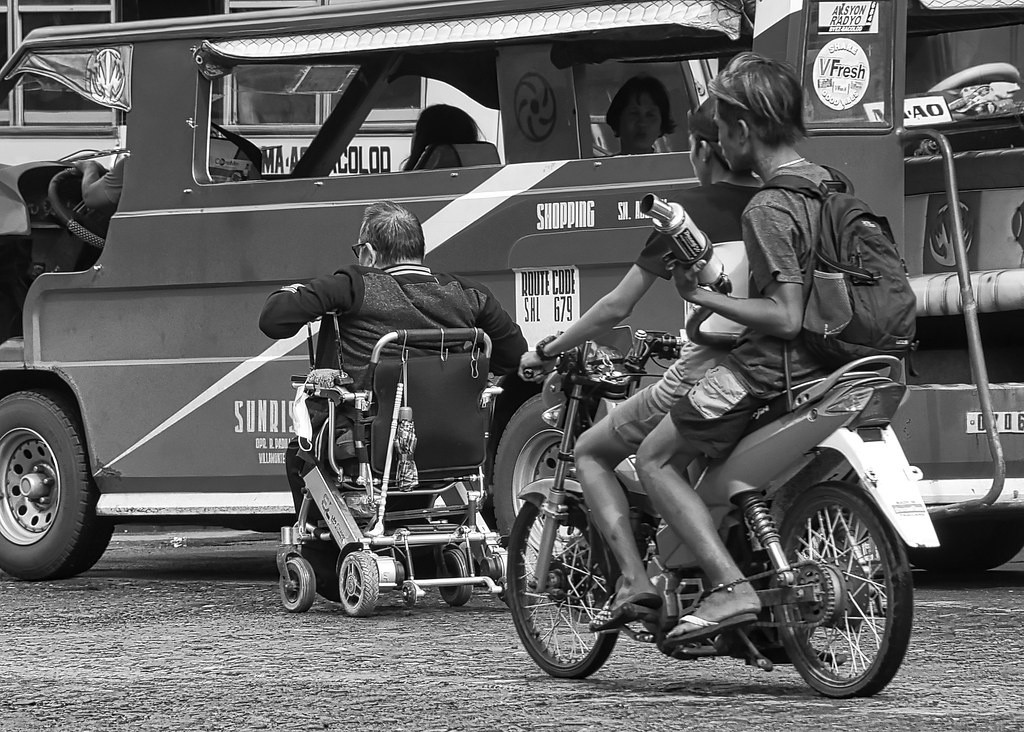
[506,329,942,698]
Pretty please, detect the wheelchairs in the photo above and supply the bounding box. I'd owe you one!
[277,310,511,618]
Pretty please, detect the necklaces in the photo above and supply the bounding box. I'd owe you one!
[778,158,805,168]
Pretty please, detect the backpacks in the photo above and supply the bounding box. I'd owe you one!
[765,163,918,371]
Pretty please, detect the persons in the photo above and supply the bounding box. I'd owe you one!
[259,200,528,603]
[399,104,478,172]
[635,52,855,647]
[606,75,675,156]
[518,97,764,633]
[76,158,125,209]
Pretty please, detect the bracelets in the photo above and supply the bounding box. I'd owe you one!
[536,336,561,362]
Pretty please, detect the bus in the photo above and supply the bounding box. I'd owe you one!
[1,0,754,583]
[752,0,1024,576]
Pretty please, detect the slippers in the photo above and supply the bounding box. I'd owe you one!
[589,591,662,631]
[665,608,759,643]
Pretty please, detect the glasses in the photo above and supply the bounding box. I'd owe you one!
[351,244,365,258]
